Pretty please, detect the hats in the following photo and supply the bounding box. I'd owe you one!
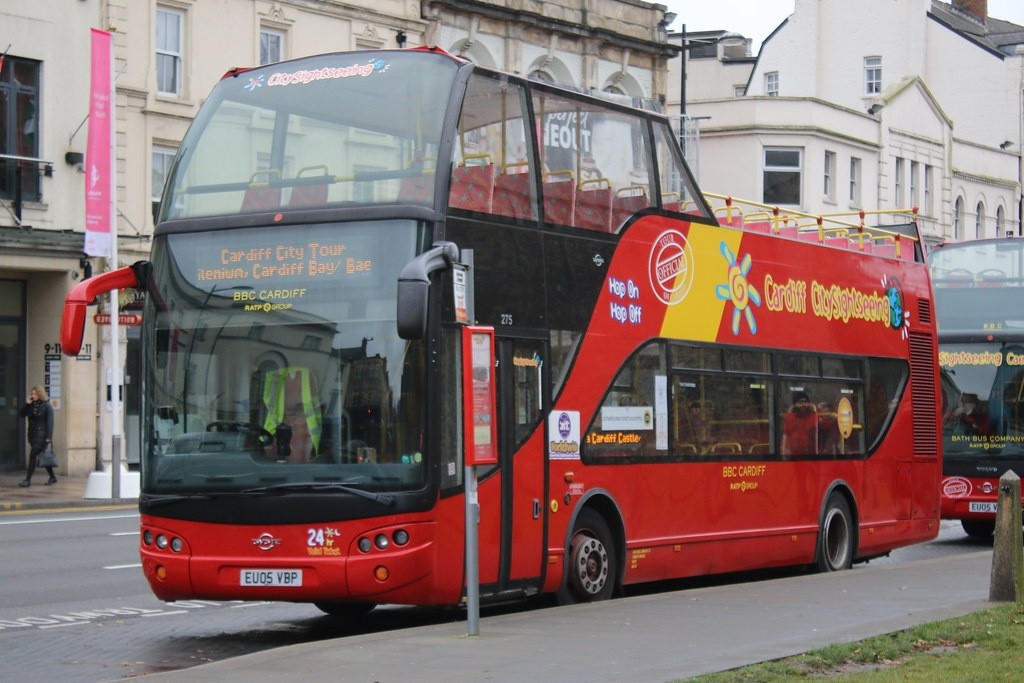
[790,391,808,405]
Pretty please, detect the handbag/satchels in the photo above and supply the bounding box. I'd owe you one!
[30,441,58,467]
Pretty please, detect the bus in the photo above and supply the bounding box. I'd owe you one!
[923,235,1024,543]
[59,48,943,619]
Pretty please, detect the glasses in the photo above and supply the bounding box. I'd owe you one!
[796,398,808,404]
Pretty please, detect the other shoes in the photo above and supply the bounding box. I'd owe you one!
[44,477,57,486]
[18,480,31,487]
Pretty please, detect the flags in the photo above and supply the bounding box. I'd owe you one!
[81,29,114,261]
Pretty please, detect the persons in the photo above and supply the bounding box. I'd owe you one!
[944,386,990,440]
[818,401,847,455]
[782,390,839,458]
[19,385,58,488]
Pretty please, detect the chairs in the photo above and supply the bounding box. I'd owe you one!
[242,161,903,261]
[657,393,856,461]
